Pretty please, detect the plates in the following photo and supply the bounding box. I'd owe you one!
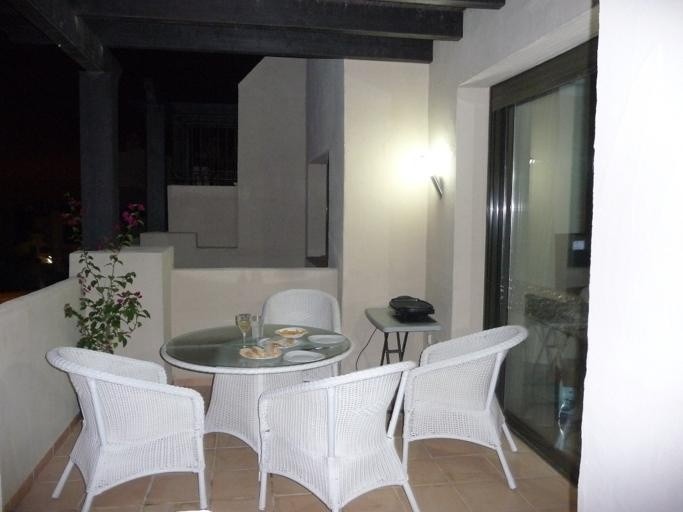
[239,327,345,363]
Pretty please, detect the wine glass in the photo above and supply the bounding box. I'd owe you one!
[235,313,250,348]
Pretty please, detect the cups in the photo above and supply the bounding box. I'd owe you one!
[250,316,263,346]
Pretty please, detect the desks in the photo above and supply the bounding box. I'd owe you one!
[518,312,588,429]
[365,307,442,367]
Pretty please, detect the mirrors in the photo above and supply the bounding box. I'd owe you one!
[306,150,329,267]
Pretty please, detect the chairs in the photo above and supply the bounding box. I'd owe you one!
[388,324,529,488]
[260,289,343,333]
[256,361,420,511]
[47,346,207,512]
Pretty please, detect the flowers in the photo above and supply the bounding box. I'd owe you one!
[52,184,147,355]
